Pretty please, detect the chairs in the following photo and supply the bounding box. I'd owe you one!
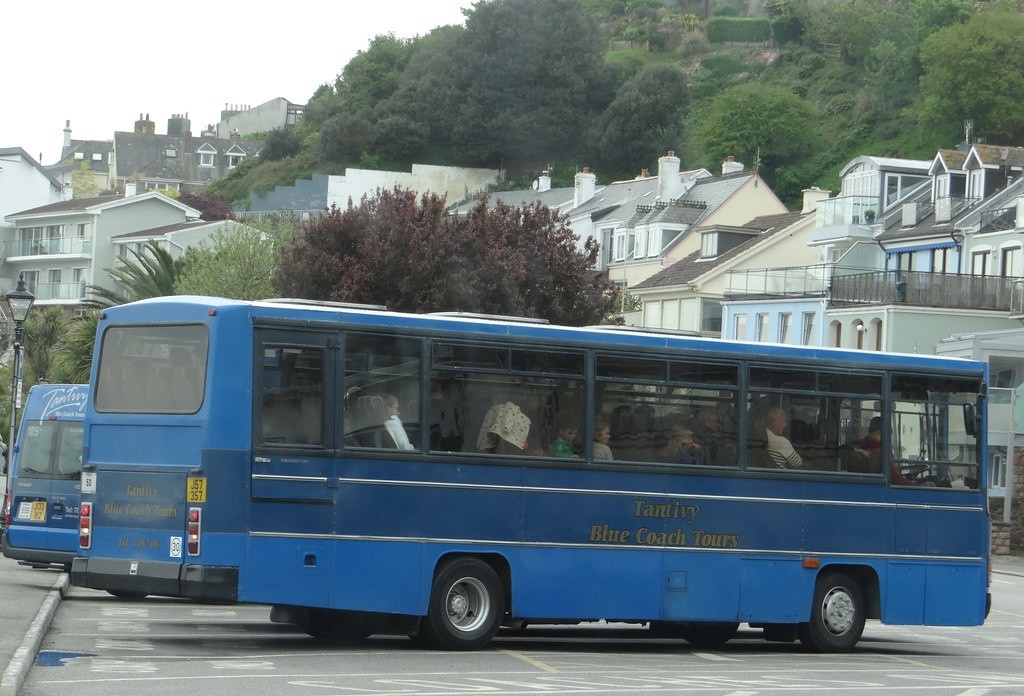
[103,347,201,409]
[351,393,878,472]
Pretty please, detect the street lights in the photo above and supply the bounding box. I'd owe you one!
[1,272,37,536]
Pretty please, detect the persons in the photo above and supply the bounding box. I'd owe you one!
[380,372,659,470]
[661,396,937,490]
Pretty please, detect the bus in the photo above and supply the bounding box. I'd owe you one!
[1,381,94,573]
[67,294,998,654]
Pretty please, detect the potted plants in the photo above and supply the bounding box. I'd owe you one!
[864,210,875,224]
